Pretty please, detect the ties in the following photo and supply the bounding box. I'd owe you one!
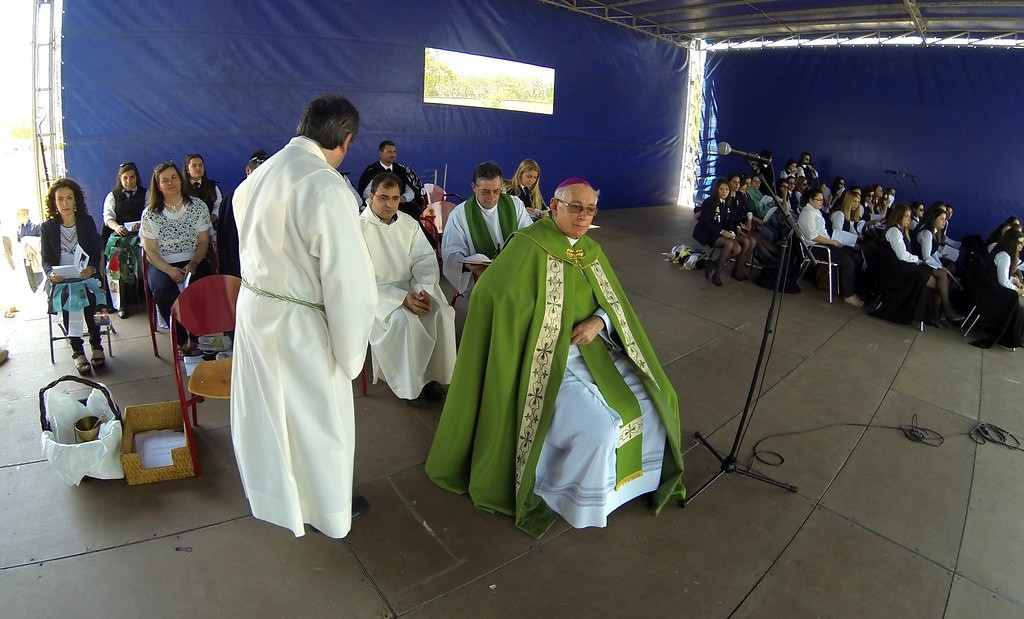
[387,168,391,174]
[124,189,136,200]
[194,182,200,197]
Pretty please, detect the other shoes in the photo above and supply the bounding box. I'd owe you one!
[842,296,864,307]
[711,271,723,287]
[739,270,749,280]
[849,294,864,304]
[731,268,743,281]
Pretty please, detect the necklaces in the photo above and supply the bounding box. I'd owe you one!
[164,196,183,214]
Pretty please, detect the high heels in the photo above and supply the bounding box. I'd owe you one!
[939,303,965,321]
[929,317,948,328]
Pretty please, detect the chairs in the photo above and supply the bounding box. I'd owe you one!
[41,184,489,479]
[695,206,1024,349]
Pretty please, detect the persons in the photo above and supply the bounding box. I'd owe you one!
[345,141,551,407]
[40,178,106,375]
[100,150,269,361]
[699,150,1024,348]
[113,283,117,293]
[110,282,115,293]
[16,208,43,293]
[231,94,380,538]
[425,176,686,540]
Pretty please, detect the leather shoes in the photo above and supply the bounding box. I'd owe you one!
[309,495,370,533]
[117,303,129,319]
[404,388,430,409]
[423,381,446,401]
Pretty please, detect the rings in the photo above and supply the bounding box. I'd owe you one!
[177,280,178,281]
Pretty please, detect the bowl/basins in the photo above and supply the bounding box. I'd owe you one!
[75,416,101,441]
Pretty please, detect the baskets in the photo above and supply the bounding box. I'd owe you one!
[39,374,124,481]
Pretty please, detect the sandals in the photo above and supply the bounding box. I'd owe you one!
[71,350,91,375]
[178,348,191,360]
[190,342,203,356]
[91,347,106,368]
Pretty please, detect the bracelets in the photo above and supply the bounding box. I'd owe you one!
[190,260,199,267]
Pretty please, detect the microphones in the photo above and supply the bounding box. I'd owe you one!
[717,142,771,164]
[902,174,916,177]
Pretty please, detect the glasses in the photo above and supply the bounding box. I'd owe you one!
[554,197,598,217]
[475,185,501,194]
[248,154,269,164]
[812,197,824,201]
[374,193,401,202]
[119,162,135,167]
[1016,240,1023,244]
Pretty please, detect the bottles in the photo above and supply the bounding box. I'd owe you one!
[156,303,169,328]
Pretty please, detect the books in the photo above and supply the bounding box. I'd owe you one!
[52,244,90,279]
[177,272,191,292]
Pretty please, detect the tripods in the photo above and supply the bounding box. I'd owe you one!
[680,158,817,507]
